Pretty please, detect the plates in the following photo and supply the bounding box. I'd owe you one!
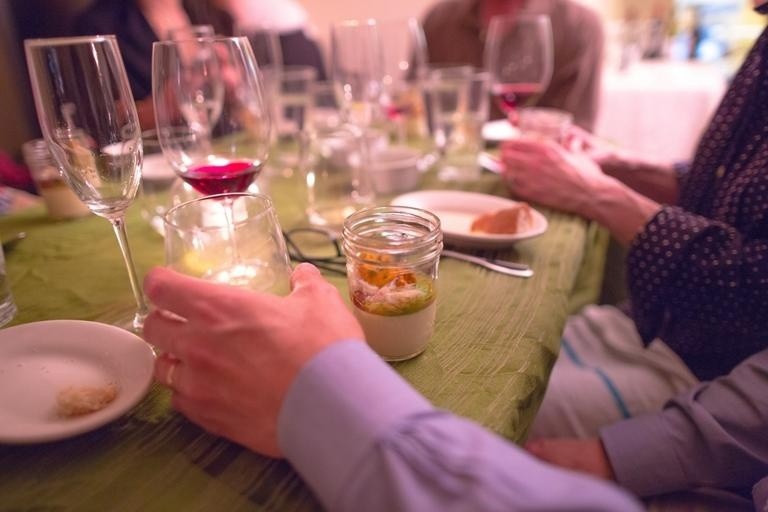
[395,188,550,248]
[2,319,156,447]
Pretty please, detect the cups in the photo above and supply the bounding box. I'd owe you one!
[162,191,296,302]
[164,25,224,147]
[25,136,100,222]
[430,13,554,181]
[233,19,429,173]
[305,125,377,235]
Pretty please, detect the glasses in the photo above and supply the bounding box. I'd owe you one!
[280,226,346,279]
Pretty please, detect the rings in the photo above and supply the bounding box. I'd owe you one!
[168,361,179,387]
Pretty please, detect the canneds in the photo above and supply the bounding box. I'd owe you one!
[21,138,94,221]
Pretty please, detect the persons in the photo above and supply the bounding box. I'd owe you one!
[498,0,768,439]
[402,1,606,148]
[143,261,768,510]
[0,1,338,196]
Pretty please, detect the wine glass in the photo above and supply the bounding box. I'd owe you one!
[26,33,154,339]
[151,35,277,298]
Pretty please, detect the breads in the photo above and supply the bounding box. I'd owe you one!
[471,204,533,235]
